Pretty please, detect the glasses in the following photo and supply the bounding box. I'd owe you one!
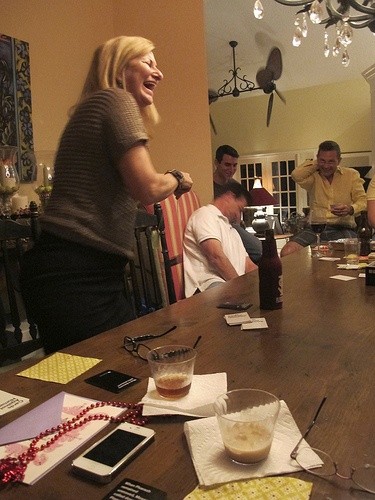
[123,325,202,362]
[290,395,375,494]
[318,159,338,166]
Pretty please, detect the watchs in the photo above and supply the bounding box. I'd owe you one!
[164,169,184,194]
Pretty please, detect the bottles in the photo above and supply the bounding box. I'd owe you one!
[258,229,283,310]
[357,211,372,256]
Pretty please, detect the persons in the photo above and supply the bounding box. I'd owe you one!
[213,144,262,262]
[279,141,366,257]
[366,175,375,229]
[182,183,258,298]
[33,36,193,353]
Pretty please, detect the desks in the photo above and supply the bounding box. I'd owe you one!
[0,240,375,500]
[258,234,294,243]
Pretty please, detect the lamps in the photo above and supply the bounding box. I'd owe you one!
[243,176,280,237]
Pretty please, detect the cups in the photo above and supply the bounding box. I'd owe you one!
[146,345,197,399]
[331,202,340,213]
[213,389,281,465]
[345,241,362,266]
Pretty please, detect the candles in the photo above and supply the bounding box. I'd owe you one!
[43,164,48,186]
[3,164,15,188]
[12,192,28,210]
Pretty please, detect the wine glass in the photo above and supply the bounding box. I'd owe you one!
[310,209,327,256]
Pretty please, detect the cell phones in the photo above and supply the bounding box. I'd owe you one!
[72,422,156,483]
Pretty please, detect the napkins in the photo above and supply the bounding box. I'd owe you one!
[337,263,361,270]
[224,311,269,331]
[312,245,327,251]
[359,273,365,277]
[135,372,324,500]
[317,256,341,262]
[16,352,104,385]
[329,274,358,281]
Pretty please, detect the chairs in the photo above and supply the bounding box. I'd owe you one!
[0,201,40,367]
[122,201,177,319]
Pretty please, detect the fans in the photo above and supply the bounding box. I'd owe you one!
[208,41,287,135]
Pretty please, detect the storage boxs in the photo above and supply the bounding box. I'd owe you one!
[365,261,375,286]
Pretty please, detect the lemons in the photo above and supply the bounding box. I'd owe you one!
[38,186,53,193]
[0,185,18,194]
